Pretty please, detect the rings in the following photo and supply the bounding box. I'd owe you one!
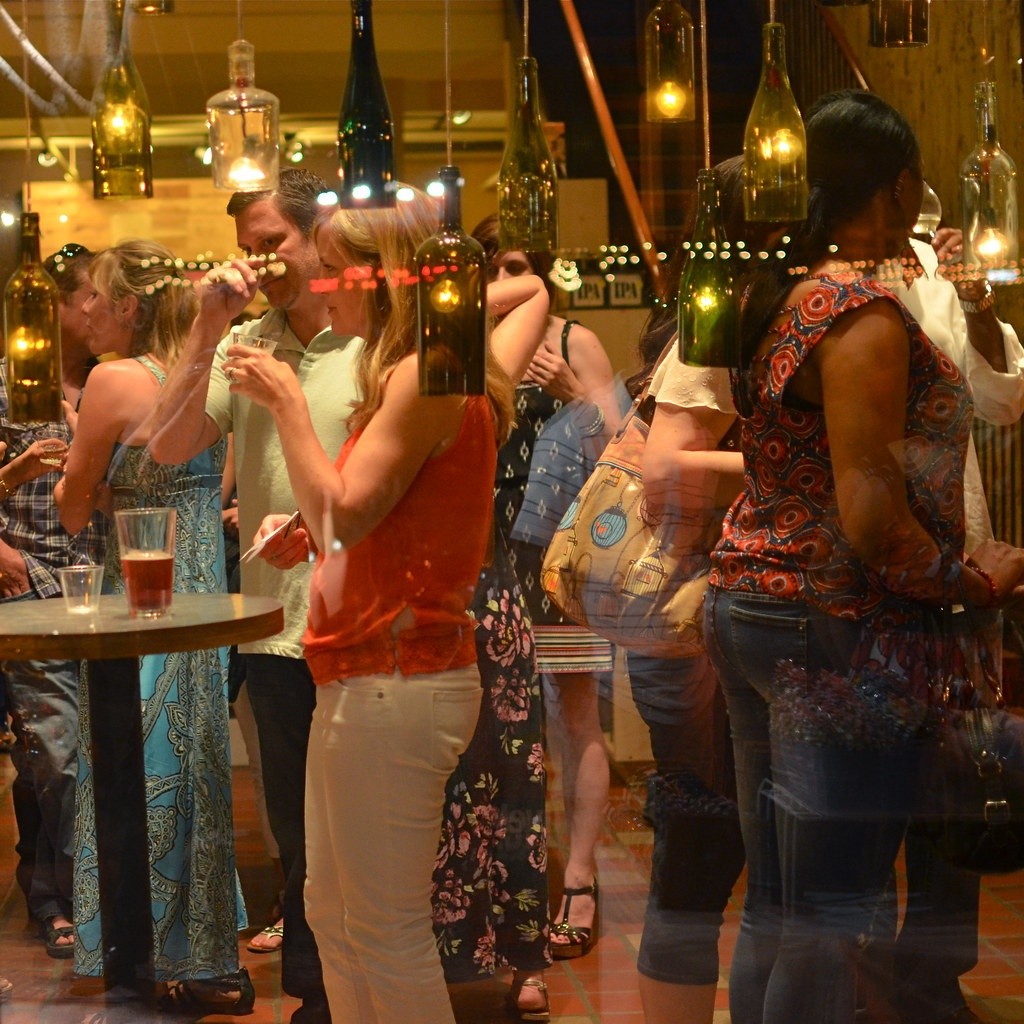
[213,273,221,284]
[230,368,236,381]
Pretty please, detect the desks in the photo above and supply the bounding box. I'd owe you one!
[0,591,284,1024]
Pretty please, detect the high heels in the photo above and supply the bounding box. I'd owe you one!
[549,875,599,958]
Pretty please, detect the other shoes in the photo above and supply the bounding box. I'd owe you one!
[0,978,12,993]
[867,950,981,1024]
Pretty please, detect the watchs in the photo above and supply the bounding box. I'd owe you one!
[958,280,997,313]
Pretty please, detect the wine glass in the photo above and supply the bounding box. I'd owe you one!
[912,179,964,281]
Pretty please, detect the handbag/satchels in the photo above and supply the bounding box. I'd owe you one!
[915,599,1024,875]
[538,331,746,661]
[511,400,604,546]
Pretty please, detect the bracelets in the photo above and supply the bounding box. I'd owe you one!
[967,567,997,616]
[571,403,606,441]
[0,479,17,496]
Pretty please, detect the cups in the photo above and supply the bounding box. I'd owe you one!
[55,564,107,615]
[114,506,184,623]
[232,331,278,356]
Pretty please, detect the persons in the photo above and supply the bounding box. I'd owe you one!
[0,242,113,958]
[53,239,255,1021]
[434,275,553,1024]
[626,98,1024,1024]
[467,210,623,958]
[149,169,369,1024]
[221,180,497,1024]
[0,438,70,501]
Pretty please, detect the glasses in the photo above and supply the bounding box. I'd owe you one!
[48,243,88,273]
[487,260,528,277]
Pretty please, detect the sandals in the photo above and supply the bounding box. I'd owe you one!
[155,966,255,1015]
[506,979,551,1021]
[247,918,284,952]
[43,917,75,956]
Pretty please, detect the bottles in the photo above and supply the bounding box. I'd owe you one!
[88,0,155,202]
[743,22,810,224]
[3,212,63,426]
[676,169,743,371]
[333,0,399,210]
[645,0,699,125]
[958,80,1022,284]
[496,57,562,276]
[411,166,489,399]
